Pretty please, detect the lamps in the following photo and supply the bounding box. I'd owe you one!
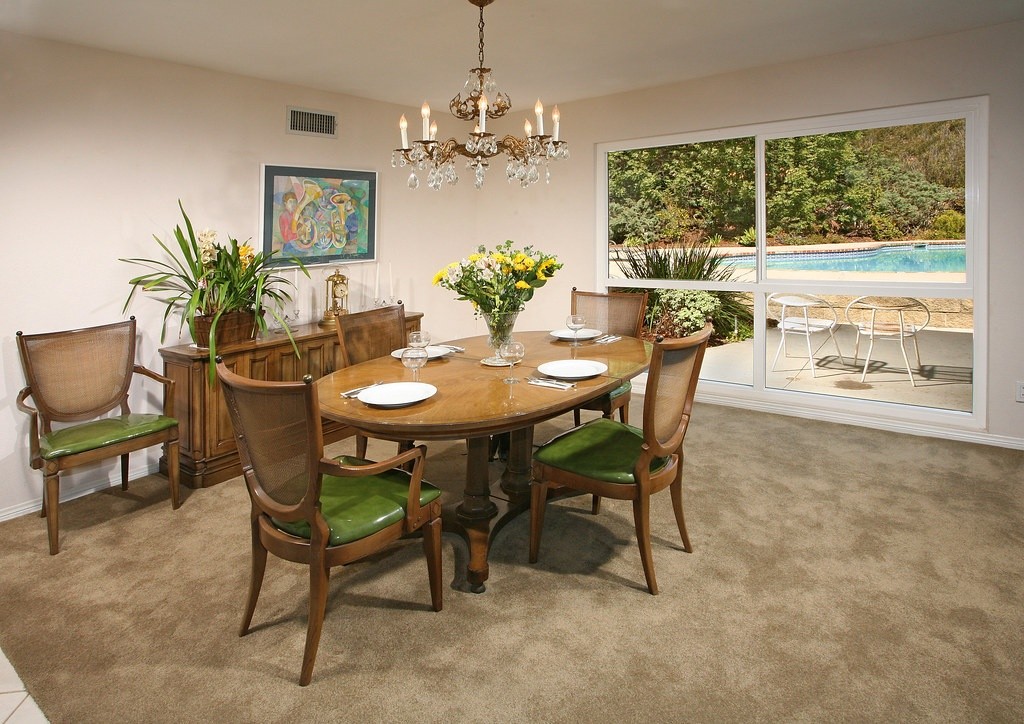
[392,0,570,191]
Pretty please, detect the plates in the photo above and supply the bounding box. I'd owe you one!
[538,360,608,379]
[391,346,451,360]
[550,328,602,341]
[358,382,437,408]
[480,356,522,367]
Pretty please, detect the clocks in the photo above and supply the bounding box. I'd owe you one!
[318,268,352,326]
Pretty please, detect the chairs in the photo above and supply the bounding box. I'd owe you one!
[570,287,648,425]
[766,293,844,377]
[332,304,418,473]
[845,296,931,388]
[528,322,713,596]
[15,315,179,556]
[214,356,443,685]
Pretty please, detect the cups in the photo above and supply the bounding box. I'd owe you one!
[408,331,431,348]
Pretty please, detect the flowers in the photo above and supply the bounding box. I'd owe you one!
[432,240,564,314]
[118,198,312,385]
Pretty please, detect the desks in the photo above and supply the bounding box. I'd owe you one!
[159,310,424,489]
[313,332,654,593]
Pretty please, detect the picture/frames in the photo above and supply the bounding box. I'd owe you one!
[258,163,378,271]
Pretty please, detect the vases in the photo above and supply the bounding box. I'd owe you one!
[481,310,521,367]
[186,308,266,347]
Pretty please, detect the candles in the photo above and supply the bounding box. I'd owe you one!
[374,261,380,299]
[276,271,281,304]
[389,261,393,298]
[294,269,298,310]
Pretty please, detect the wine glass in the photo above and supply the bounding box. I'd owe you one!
[501,342,524,385]
[401,348,428,382]
[567,314,586,347]
